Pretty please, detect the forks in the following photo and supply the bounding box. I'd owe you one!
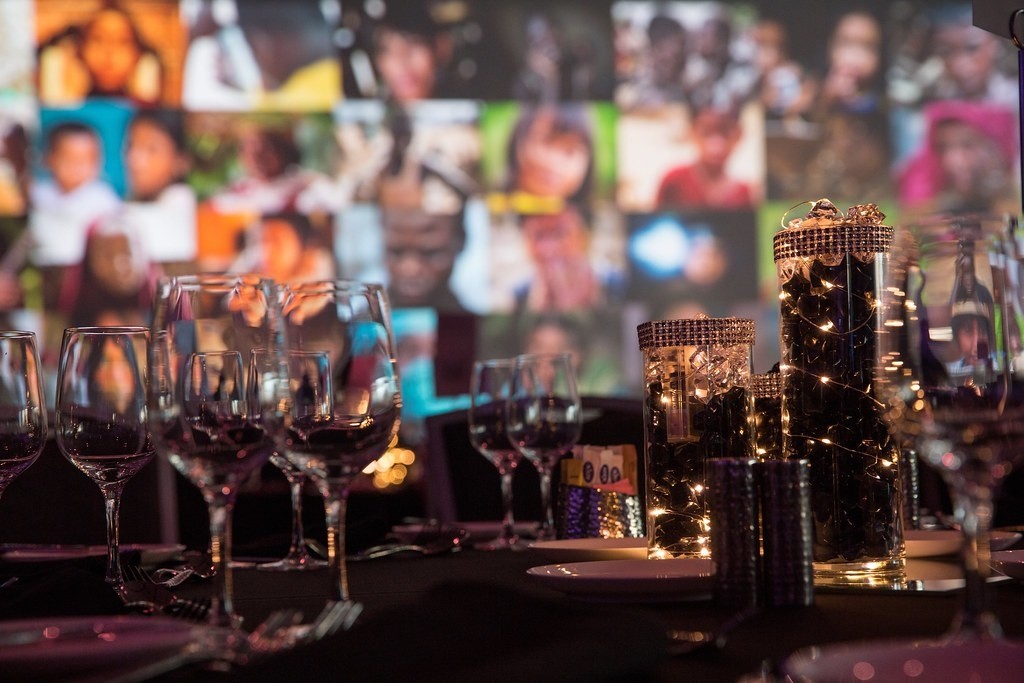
[361,521,471,561]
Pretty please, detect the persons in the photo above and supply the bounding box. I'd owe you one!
[0,2,1019,427]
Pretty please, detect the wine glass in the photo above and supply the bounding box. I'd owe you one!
[54,275,402,640]
[505,352,584,552]
[0,330,48,588]
[469,359,523,551]
[876,214,1024,638]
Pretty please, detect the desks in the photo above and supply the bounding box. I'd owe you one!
[0,542,1024,683]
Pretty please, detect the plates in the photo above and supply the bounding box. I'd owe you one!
[525,559,716,603]
[0,616,206,683]
[529,537,649,561]
[992,550,1024,579]
[905,528,1021,557]
[784,639,1024,683]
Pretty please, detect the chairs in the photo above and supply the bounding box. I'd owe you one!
[426,396,647,523]
